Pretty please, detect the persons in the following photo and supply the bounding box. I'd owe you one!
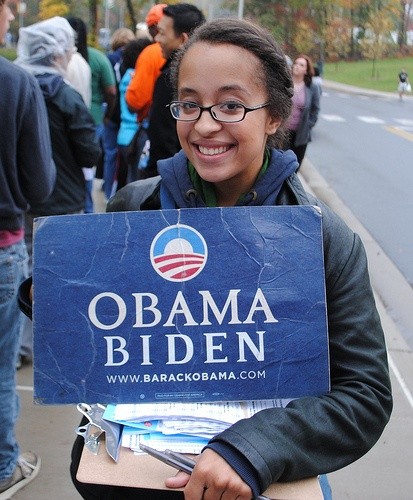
[398,68,408,98]
[16,18,393,500]
[0,1,57,500]
[282,54,323,173]
[66,3,207,212]
[13,16,102,367]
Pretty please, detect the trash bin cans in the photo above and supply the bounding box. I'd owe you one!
[317,62,322,73]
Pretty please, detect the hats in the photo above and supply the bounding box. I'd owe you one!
[145,3,169,27]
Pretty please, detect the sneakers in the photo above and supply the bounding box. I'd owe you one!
[0,452,42,500]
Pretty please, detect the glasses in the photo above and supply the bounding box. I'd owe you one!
[165,99,271,123]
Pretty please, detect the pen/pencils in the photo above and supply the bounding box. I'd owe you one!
[165,449,271,500]
[139,443,193,475]
